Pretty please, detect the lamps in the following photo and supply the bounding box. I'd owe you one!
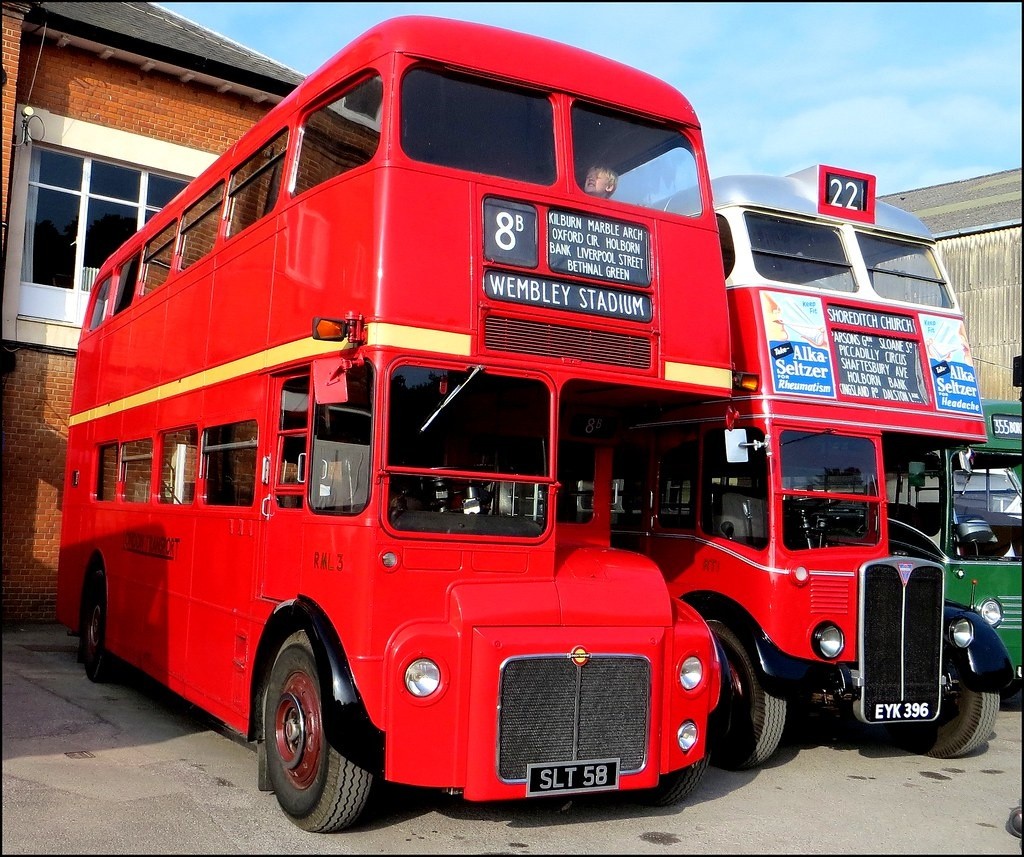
[21,106,35,122]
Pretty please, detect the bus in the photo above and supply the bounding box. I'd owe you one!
[867,399,1022,700]
[482,164,1014,774]
[54,15,741,838]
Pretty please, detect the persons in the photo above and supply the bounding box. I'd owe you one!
[388,476,423,522]
[584,165,618,198]
